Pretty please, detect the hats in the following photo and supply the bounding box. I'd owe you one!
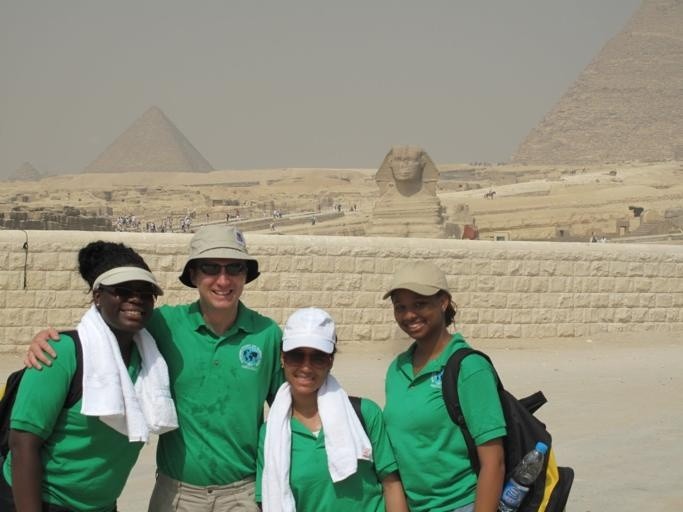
[91,267,165,297]
[178,224,261,289]
[382,260,452,300]
[282,306,339,356]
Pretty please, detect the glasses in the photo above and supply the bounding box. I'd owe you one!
[201,263,245,277]
[114,287,155,302]
[282,349,332,371]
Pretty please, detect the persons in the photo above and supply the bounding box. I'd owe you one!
[116,205,356,231]
[0,242,164,512]
[382,262,508,512]
[254,308,410,512]
[375,143,438,200]
[24,225,283,512]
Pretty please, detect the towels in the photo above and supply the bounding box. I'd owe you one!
[76,302,178,443]
[259,370,374,511]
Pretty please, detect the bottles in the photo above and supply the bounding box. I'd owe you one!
[497,442,549,511]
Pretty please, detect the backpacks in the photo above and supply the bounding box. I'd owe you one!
[441,343,577,512]
[0,329,90,454]
